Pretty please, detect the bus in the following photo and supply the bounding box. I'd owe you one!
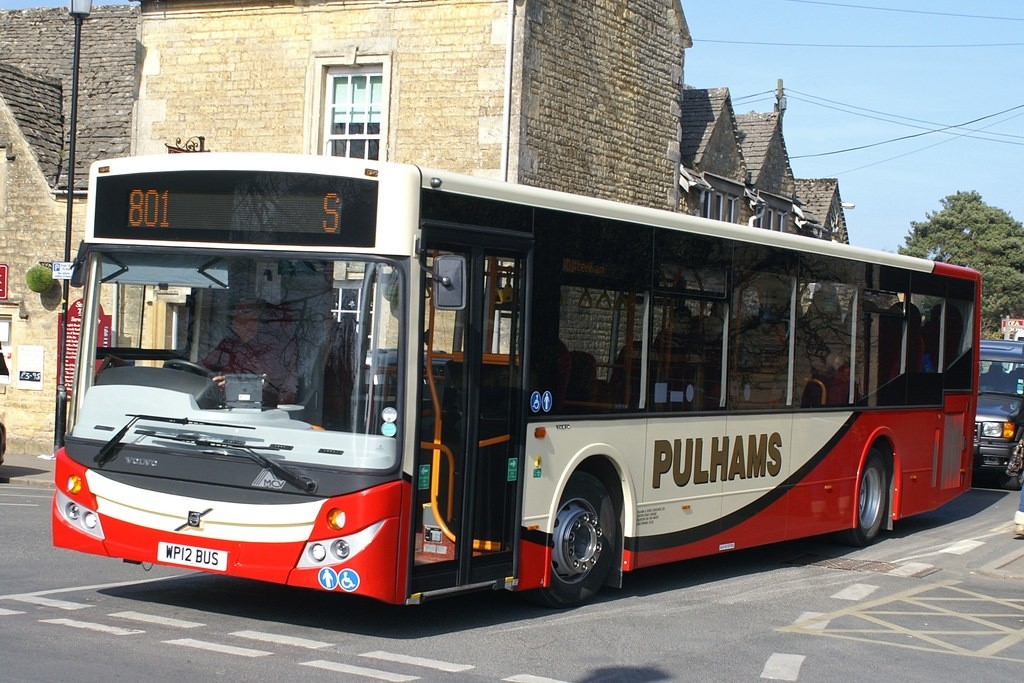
[51,152,984,608]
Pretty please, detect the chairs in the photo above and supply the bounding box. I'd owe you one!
[979,363,1024,392]
[377,296,964,413]
[283,273,333,423]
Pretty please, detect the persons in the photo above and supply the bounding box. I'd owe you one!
[192,291,299,406]
[652,281,842,410]
[1009,438,1024,540]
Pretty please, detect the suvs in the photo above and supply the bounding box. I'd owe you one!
[973,339,1024,489]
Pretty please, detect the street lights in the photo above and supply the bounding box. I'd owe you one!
[53,0,93,453]
